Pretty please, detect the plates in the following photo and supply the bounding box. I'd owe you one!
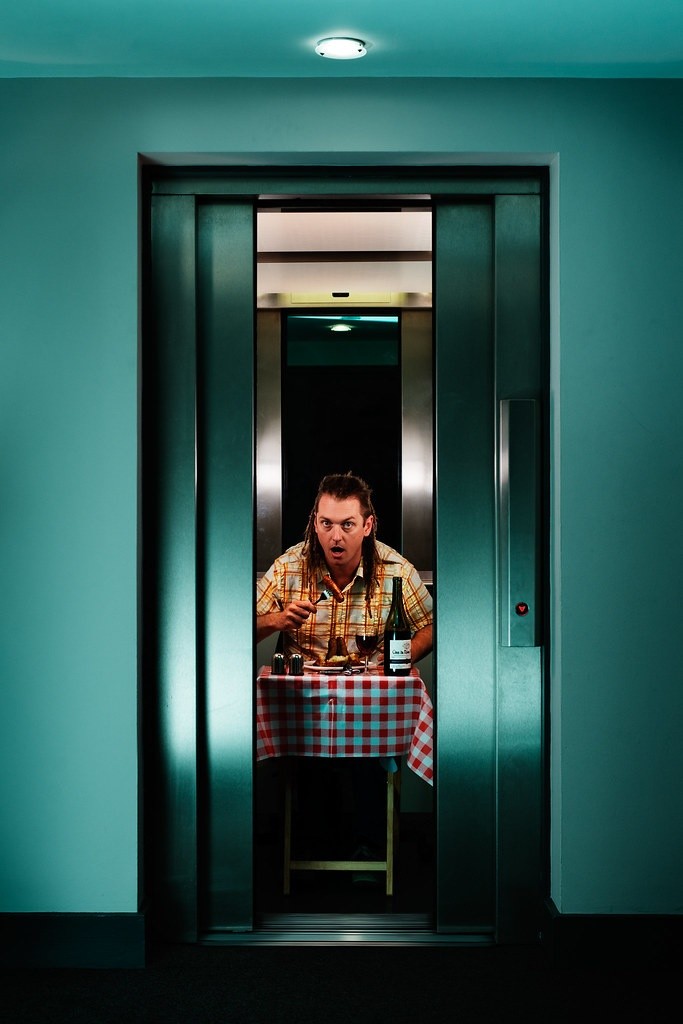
[303,660,377,670]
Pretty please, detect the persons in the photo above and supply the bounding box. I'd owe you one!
[256,471,434,892]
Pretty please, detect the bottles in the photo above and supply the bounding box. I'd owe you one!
[271,653,286,675]
[384,576,412,677]
[289,653,303,676]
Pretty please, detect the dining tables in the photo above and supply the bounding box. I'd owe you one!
[257,666,434,896]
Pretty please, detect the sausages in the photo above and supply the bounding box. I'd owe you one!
[322,576,344,603]
[326,635,348,660]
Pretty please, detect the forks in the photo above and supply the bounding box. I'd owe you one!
[313,589,333,606]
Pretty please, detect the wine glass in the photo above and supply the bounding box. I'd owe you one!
[356,627,377,676]
